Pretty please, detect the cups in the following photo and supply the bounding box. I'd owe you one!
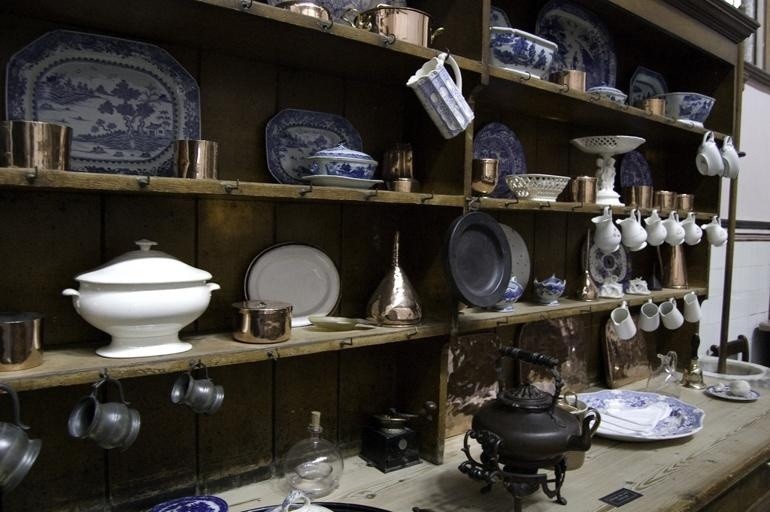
[489,276,524,313]
[534,273,566,305]
[573,176,694,211]
[407,53,477,139]
[175,138,220,180]
[392,178,421,193]
[1,120,73,172]
[557,391,601,470]
[610,290,702,340]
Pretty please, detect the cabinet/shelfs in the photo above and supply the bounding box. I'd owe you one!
[0,0,769,512]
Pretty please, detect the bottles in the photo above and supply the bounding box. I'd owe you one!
[0,310,45,372]
[641,245,689,290]
[369,230,423,325]
[285,411,344,495]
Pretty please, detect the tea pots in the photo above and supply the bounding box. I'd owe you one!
[68,377,141,454]
[591,207,728,254]
[696,131,740,178]
[0,384,44,500]
[171,363,224,415]
[473,345,599,468]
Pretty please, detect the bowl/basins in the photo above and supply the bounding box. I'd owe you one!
[62,239,221,358]
[489,2,628,105]
[630,67,716,128]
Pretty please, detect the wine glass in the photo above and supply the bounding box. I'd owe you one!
[472,158,499,198]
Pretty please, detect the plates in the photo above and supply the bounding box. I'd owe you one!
[705,385,761,402]
[146,495,227,512]
[5,29,202,178]
[473,123,528,199]
[265,108,384,190]
[444,211,531,307]
[620,151,653,193]
[244,242,368,329]
[568,389,705,443]
[590,243,627,283]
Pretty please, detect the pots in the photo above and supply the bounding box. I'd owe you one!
[232,299,294,344]
[268,0,444,48]
